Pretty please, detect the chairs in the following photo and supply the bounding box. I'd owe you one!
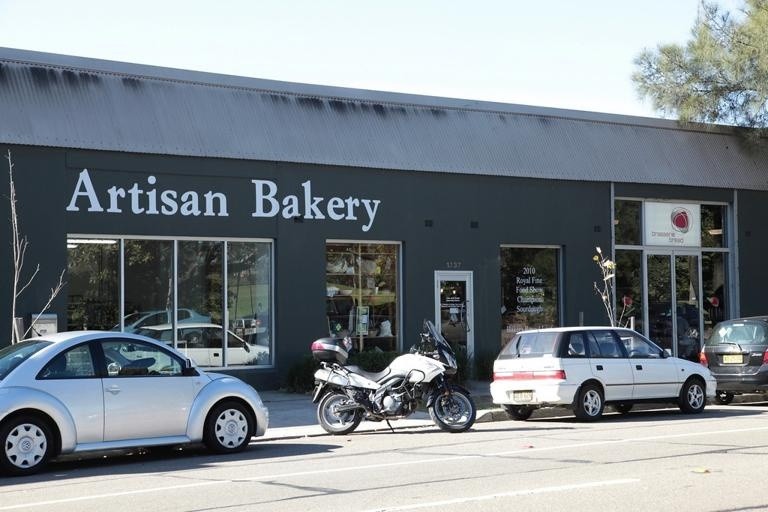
[571,342,615,357]
[45,355,73,379]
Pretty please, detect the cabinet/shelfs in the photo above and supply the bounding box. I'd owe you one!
[326,243,398,352]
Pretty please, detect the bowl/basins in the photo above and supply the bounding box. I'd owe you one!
[340,289,353,295]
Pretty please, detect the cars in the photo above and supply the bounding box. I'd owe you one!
[0,331,268,476]
[489,315,768,422]
[111,308,271,363]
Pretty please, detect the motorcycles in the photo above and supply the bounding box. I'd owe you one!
[311,319,476,435]
